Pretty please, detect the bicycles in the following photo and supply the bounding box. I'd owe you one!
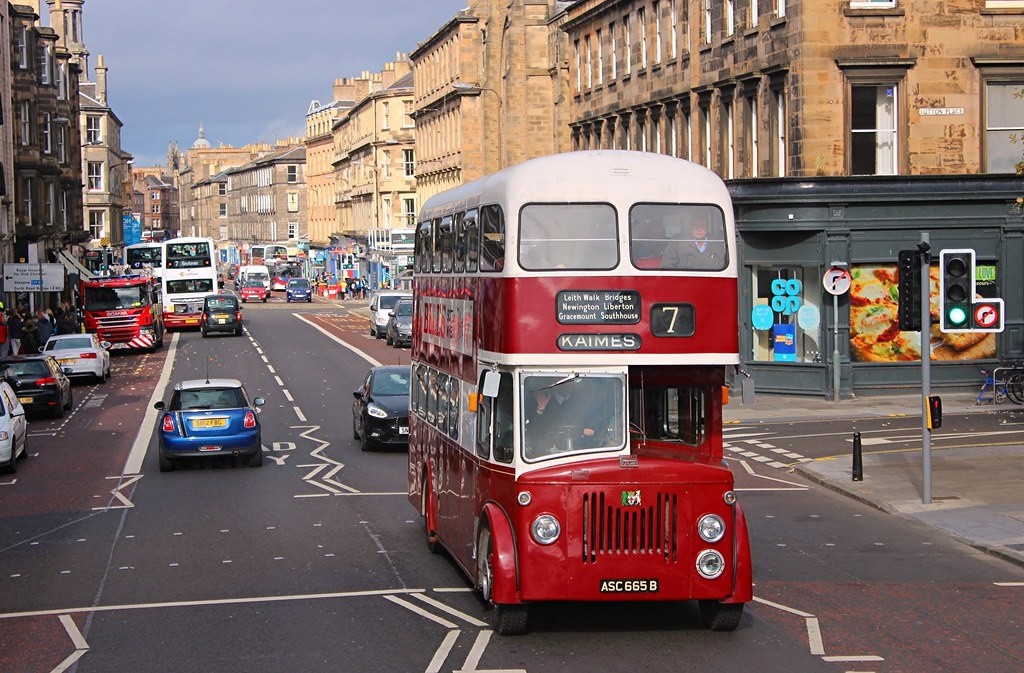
[1003,356,1024,405]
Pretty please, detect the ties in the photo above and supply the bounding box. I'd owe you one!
[562,399,568,406]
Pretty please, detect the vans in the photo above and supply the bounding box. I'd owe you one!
[370,291,413,340]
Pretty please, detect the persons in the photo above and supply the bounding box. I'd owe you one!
[310,271,369,302]
[0,298,76,359]
[528,377,612,447]
[263,276,268,280]
[364,381,371,397]
[247,284,252,288]
[661,214,725,270]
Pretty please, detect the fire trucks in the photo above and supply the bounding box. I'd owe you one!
[78,275,163,353]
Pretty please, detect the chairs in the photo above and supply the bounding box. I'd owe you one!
[182,393,199,406]
[637,257,662,267]
[383,304,392,309]
[210,301,215,305]
[227,301,231,303]
[33,364,43,374]
[494,258,504,272]
[218,391,236,408]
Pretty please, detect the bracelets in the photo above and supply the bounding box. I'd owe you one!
[537,409,543,411]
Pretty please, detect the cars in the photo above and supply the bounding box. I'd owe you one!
[0,378,29,472]
[240,281,269,302]
[227,263,248,290]
[0,353,73,420]
[284,278,314,302]
[352,366,412,452]
[386,297,414,348]
[154,378,267,471]
[38,333,113,383]
[197,295,243,336]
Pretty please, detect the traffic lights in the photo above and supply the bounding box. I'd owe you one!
[931,394,942,428]
[939,247,976,333]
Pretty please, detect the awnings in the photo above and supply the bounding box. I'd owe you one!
[53,249,96,283]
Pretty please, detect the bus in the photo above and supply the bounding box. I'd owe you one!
[123,241,163,300]
[265,259,304,291]
[162,236,218,331]
[250,244,288,275]
[406,148,754,633]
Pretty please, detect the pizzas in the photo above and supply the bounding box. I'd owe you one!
[848,266,996,362]
